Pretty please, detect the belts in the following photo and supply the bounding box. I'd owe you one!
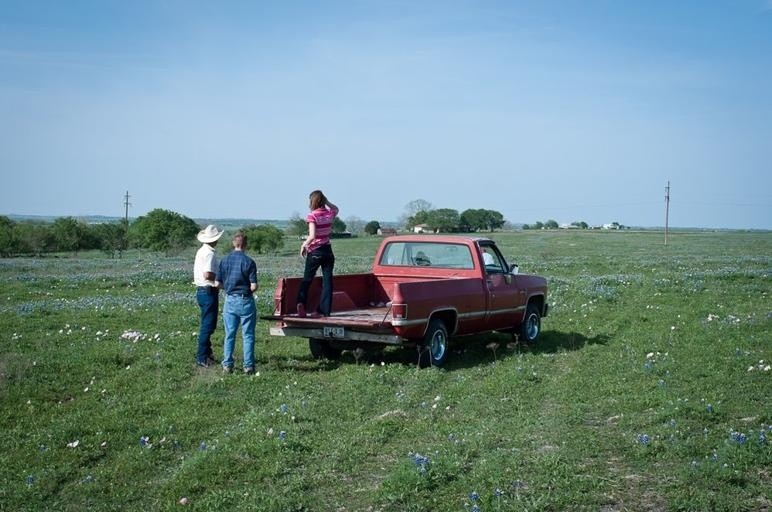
[230,293,251,298]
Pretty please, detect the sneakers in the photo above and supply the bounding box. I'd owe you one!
[311,311,330,317]
[243,366,255,373]
[222,366,232,373]
[197,356,217,367]
[297,303,306,318]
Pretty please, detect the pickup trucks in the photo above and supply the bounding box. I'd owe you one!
[258,232,550,367]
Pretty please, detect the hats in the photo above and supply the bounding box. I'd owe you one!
[197,224,225,243]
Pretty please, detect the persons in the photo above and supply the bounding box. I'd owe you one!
[191,223,225,369]
[214,233,260,375]
[295,190,339,319]
